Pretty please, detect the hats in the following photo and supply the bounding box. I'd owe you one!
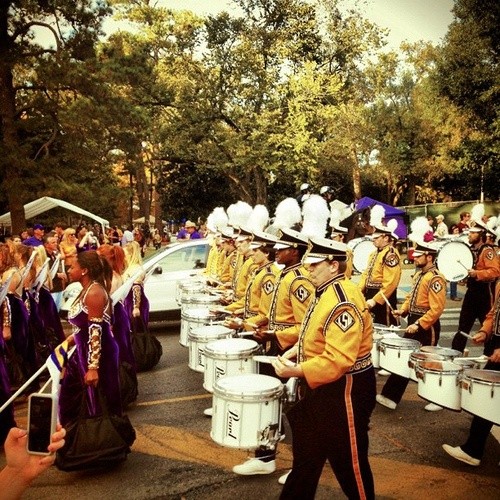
[435,214,444,220]
[449,224,459,233]
[301,235,348,264]
[468,203,500,246]
[33,224,45,230]
[204,194,351,251]
[408,215,438,256]
[184,221,196,228]
[369,204,400,243]
[57,223,62,227]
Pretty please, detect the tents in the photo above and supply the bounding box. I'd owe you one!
[0,197,110,236]
[133,215,167,228]
[352,195,407,239]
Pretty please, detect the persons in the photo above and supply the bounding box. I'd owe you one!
[204,231,240,418]
[232,230,316,483]
[69,219,206,409]
[440,281,500,467]
[450,217,500,359]
[270,235,376,500]
[455,212,470,230]
[212,231,279,348]
[359,227,402,376]
[297,182,349,242]
[0,426,66,500]
[435,214,449,238]
[53,251,135,471]
[446,225,462,301]
[376,242,447,412]
[0,222,76,450]
[483,225,500,253]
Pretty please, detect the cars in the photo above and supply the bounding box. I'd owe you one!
[127,238,229,314]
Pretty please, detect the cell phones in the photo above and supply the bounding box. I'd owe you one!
[26,393,58,456]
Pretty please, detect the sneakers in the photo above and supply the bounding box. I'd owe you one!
[232,456,277,475]
[278,468,293,484]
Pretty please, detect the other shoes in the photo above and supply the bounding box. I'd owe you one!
[424,403,444,411]
[203,407,213,417]
[375,394,398,410]
[452,296,462,301]
[377,368,392,375]
[442,442,481,466]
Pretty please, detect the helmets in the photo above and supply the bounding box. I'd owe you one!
[320,186,334,195]
[300,183,313,192]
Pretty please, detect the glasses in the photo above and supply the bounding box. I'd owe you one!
[39,228,45,231]
[69,234,76,236]
